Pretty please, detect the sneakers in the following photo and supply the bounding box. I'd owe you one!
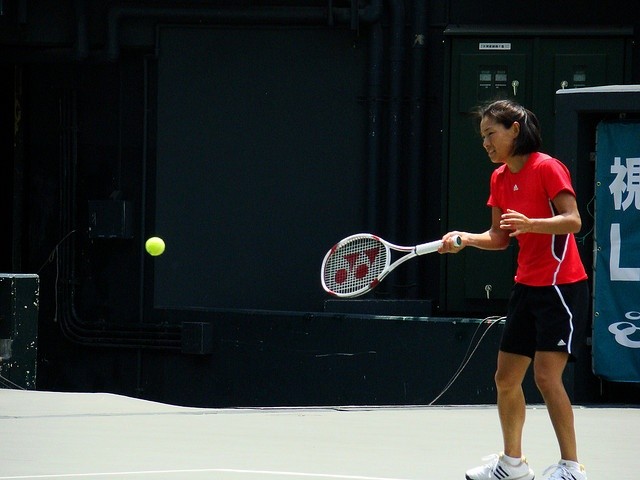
[465,453,535,480]
[542,458,587,480]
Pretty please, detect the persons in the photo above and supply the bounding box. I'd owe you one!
[437,98,589,480]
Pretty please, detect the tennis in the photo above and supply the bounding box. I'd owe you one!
[145,237,165,256]
